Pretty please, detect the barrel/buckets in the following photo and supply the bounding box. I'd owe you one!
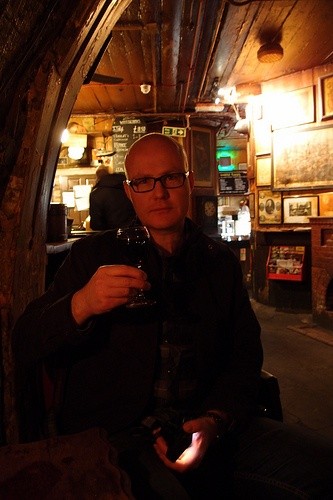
[46,204,68,244]
[62,184,93,211]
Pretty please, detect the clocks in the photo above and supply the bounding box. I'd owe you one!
[195,195,219,235]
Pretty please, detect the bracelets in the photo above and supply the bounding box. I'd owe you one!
[205,413,226,443]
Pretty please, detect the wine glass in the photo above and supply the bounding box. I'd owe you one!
[115,226,156,308]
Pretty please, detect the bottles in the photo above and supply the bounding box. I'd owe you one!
[216,199,251,242]
[86,212,93,231]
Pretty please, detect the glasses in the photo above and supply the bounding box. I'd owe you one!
[126,171,189,193]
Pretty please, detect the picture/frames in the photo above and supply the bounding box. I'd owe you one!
[258,189,282,226]
[256,156,271,187]
[282,196,320,224]
[318,72,333,121]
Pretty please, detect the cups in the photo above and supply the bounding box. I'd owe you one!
[67,219,74,234]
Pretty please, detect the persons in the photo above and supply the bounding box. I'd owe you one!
[89,166,136,231]
[12,132,264,500]
